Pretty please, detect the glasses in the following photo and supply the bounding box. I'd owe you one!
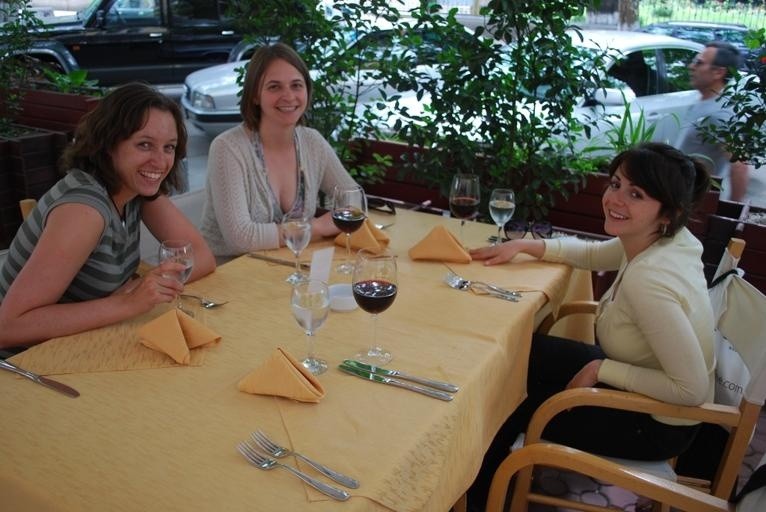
[692,56,728,69]
[367,193,400,218]
[503,214,557,243]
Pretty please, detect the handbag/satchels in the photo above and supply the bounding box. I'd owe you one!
[705,262,761,447]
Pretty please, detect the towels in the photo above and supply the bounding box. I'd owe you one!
[240,345,325,404]
[410,222,474,265]
[134,306,224,367]
[332,216,391,256]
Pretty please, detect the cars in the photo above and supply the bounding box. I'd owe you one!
[179,17,521,144]
[632,19,766,83]
[315,0,447,33]
[330,31,766,195]
[17,1,158,21]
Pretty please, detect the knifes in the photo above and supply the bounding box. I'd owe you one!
[0,356,79,399]
[342,358,463,392]
[247,250,311,271]
[336,364,454,402]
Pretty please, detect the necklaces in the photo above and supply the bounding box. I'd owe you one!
[105,187,126,230]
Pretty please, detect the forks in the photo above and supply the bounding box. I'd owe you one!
[236,439,350,500]
[250,428,362,491]
[444,272,522,303]
[180,295,230,310]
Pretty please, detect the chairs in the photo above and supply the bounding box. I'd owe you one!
[507,238,766,511]
[137,185,208,266]
[0,196,156,286]
[481,442,726,512]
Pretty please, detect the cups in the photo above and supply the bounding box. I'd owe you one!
[505,218,530,240]
[328,281,359,313]
[531,221,552,240]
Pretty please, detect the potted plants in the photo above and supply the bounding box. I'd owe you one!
[0,0,69,249]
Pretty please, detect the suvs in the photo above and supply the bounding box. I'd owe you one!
[0,1,337,98]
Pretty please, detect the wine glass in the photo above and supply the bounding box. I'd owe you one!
[289,281,331,378]
[447,174,481,245]
[281,213,313,286]
[332,184,368,274]
[157,239,194,321]
[489,188,515,247]
[351,246,398,365]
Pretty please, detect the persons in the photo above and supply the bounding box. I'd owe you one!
[0,83,217,363]
[469,141,720,512]
[200,42,364,270]
[676,44,748,201]
